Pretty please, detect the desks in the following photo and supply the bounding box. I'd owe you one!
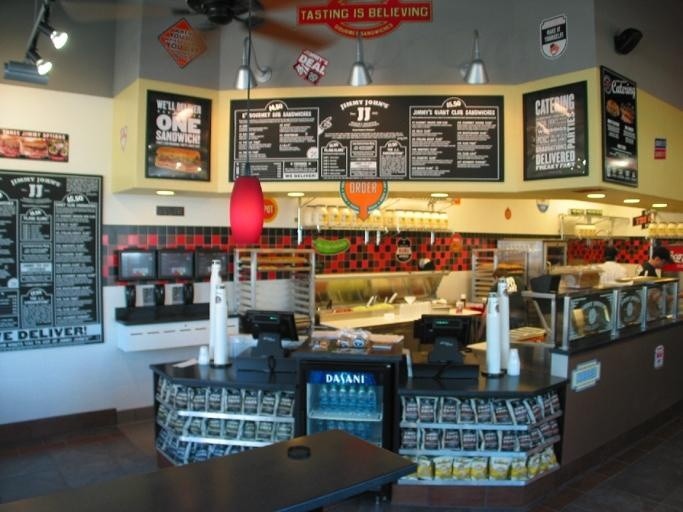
[0,427,419,512]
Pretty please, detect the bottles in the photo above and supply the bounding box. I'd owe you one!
[485,279,521,377]
[196,259,233,368]
[386,210,449,234]
[315,383,376,443]
[305,204,382,230]
[648,221,682,238]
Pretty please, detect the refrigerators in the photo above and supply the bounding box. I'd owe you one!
[295,351,396,501]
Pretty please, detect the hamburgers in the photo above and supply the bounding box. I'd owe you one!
[20,137,48,158]
[2,135,20,157]
[154,147,203,173]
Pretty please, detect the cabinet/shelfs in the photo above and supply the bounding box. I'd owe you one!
[305,360,383,450]
[233,247,316,337]
[150,361,299,470]
[398,377,563,487]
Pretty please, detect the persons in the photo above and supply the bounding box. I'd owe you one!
[474,266,529,342]
[637,247,673,277]
[599,246,626,281]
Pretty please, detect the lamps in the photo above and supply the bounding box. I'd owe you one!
[459,29,490,86]
[228,1,272,245]
[348,33,376,89]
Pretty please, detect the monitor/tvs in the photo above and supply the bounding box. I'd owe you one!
[117,250,157,281]
[156,250,193,280]
[194,249,229,279]
[246,309,298,341]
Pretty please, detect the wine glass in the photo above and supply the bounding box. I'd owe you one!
[403,295,417,316]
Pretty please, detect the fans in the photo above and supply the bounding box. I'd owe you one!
[171,0,270,35]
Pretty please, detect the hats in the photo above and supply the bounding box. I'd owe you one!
[653,247,673,265]
